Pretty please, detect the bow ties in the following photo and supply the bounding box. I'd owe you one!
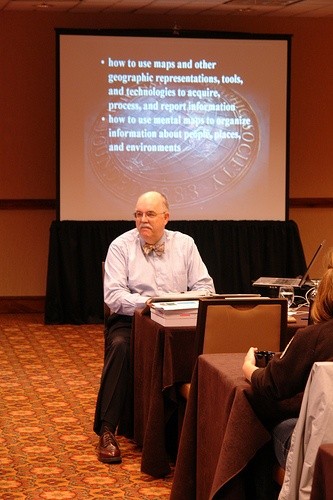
[141,242,164,258]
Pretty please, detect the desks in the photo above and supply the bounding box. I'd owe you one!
[116,300,315,479]
[169,349,282,500]
[43,216,311,325]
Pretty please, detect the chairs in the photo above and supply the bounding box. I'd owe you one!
[170,296,289,468]
[274,360,333,500]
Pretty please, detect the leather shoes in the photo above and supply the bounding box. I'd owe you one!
[94,427,122,464]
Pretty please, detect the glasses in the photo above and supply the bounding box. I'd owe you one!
[132,211,166,219]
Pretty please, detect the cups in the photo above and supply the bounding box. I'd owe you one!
[279,286,294,308]
[255,350,272,369]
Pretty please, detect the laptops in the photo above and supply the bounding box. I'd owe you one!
[253,239,326,288]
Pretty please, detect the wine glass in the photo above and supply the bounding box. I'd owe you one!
[307,280,322,307]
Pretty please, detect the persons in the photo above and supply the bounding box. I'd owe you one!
[241,268,333,471]
[92,190,216,462]
